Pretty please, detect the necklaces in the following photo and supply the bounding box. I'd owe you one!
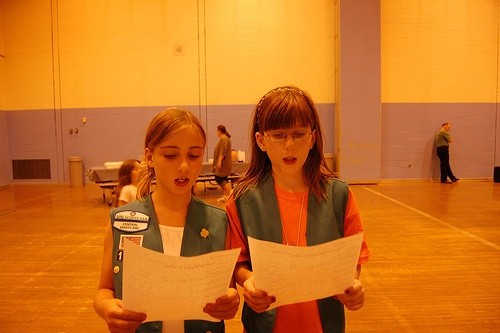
[281,190,306,247]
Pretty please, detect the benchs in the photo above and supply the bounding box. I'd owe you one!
[98,175,243,203]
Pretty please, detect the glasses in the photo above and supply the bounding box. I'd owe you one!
[263,129,312,143]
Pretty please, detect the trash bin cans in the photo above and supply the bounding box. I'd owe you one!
[67,155,84,187]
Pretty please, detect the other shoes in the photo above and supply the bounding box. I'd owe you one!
[217,195,229,202]
[451,178,460,182]
[440,180,453,184]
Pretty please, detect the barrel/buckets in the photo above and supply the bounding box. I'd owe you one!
[320,153,334,173]
[69,157,84,184]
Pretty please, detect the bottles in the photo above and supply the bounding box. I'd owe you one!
[232,150,237,162]
[238,150,243,162]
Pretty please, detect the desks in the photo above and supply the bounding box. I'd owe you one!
[89,161,249,205]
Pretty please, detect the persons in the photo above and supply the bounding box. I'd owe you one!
[115,159,142,207]
[93,107,240,333]
[224,86,371,333]
[436,123,460,184]
[212,125,233,202]
